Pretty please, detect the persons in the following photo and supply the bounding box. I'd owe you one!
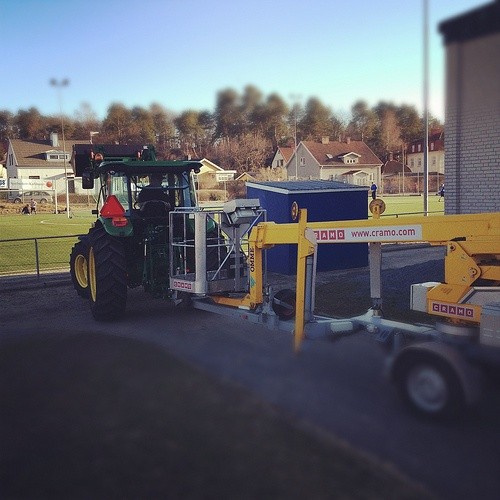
[22,204,30,215]
[137,175,170,210]
[209,194,217,201]
[371,182,378,199]
[30,199,37,214]
[438,183,444,202]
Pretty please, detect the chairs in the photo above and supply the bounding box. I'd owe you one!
[139,200,167,218]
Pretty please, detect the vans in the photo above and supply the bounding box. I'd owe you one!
[13,190,53,204]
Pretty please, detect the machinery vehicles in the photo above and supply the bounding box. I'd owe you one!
[70,142,500,416]
